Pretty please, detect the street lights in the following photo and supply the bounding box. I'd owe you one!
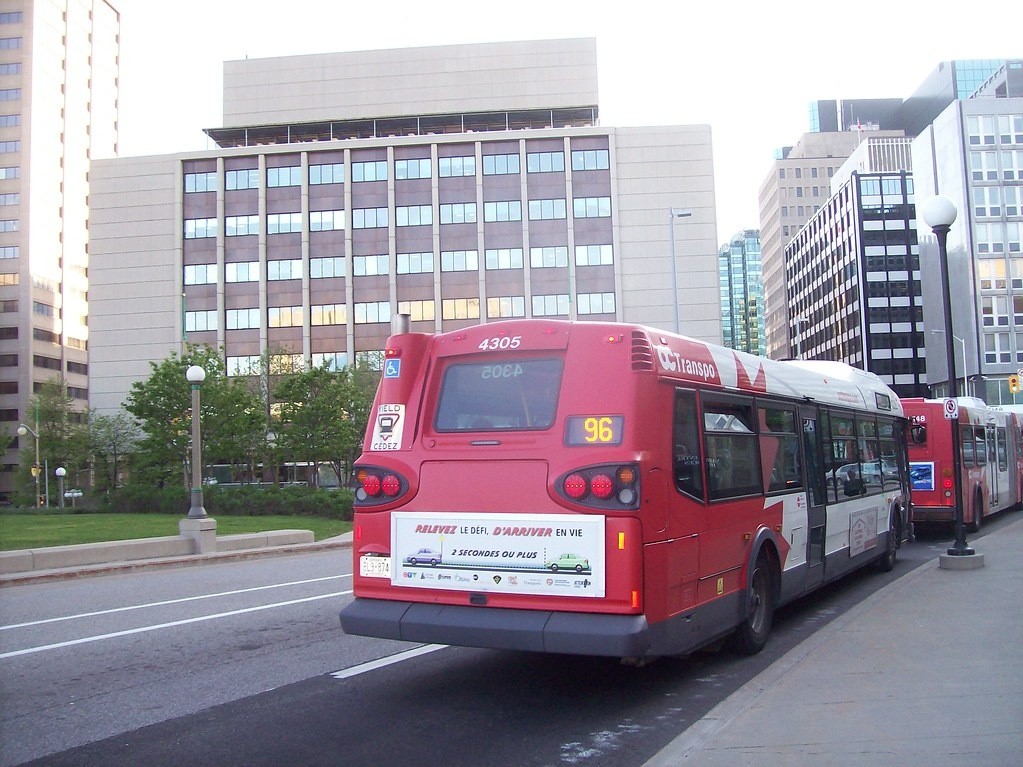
[187,365,210,521]
[667,207,692,334]
[919,196,983,572]
[16,399,41,509]
[55,467,66,510]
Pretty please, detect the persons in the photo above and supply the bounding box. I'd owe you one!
[843,470,868,496]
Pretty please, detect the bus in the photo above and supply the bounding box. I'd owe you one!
[202,461,343,493]
[339,318,930,661]
[865,396,1023,533]
